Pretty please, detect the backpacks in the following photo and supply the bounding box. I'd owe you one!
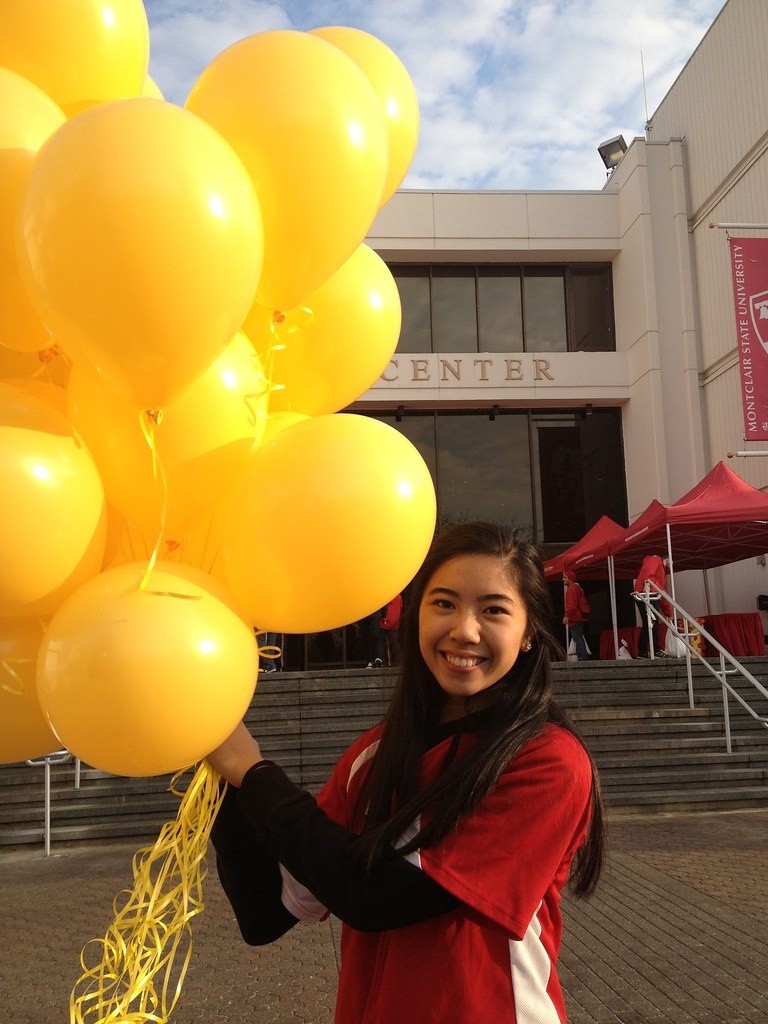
[575,585,591,614]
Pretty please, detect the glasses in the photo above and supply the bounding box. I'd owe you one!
[563,576,566,578]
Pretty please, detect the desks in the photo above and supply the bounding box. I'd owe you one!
[599,611,765,661]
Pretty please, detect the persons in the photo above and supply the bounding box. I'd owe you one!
[563,566,592,657]
[192,520,606,1024]
[380,594,403,669]
[256,633,277,674]
[633,555,669,658]
[354,611,384,669]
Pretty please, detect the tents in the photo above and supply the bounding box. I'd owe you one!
[539,514,710,658]
[606,459,768,654]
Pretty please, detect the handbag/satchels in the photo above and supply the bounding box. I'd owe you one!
[665,620,687,657]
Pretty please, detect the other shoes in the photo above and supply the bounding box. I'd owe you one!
[258,666,264,672]
[373,655,383,667]
[654,649,672,659]
[265,667,276,673]
[637,652,651,659]
[366,662,373,668]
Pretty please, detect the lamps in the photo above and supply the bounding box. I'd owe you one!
[398,406,404,415]
[492,405,499,415]
[586,403,592,413]
[597,135,627,170]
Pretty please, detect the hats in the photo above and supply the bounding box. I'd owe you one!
[565,571,577,582]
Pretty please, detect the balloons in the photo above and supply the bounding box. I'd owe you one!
[34,562,261,779]
[174,413,436,633]
[1,5,437,763]
[31,99,269,424]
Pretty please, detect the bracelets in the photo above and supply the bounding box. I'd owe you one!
[244,760,278,773]
